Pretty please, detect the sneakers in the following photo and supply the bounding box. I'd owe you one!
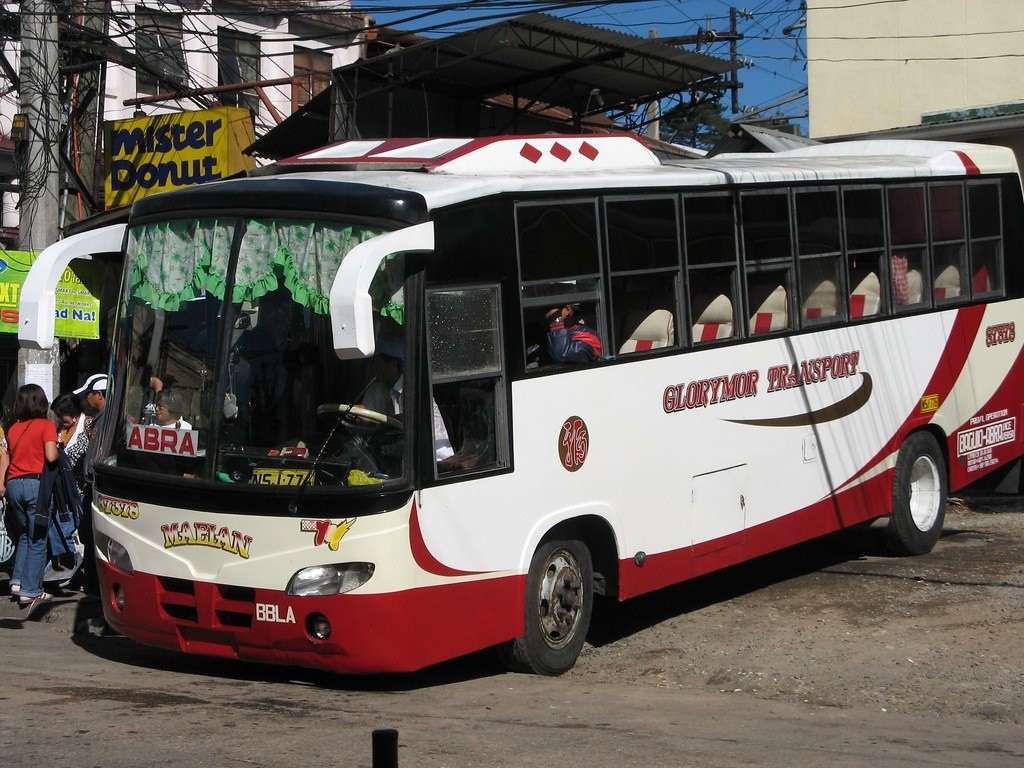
[11,584,21,596]
[20,592,53,605]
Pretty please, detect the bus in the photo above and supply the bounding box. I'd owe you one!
[16,131,1024,676]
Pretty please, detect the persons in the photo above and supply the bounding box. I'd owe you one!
[345,332,455,462]
[155,390,190,429]
[525,280,602,371]
[1,373,108,603]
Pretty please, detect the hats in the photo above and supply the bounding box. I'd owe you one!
[72,373,108,396]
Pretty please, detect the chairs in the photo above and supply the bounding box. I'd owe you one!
[903,268,923,304]
[748,284,788,335]
[849,271,881,319]
[690,293,734,343]
[615,308,674,355]
[801,277,837,321]
[935,263,961,301]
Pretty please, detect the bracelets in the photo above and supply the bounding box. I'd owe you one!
[549,317,563,323]
